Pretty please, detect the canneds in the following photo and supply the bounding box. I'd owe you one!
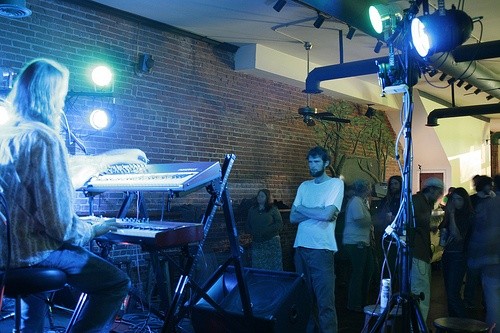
[381,278,391,308]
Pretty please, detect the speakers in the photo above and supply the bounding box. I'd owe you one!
[141,54,154,72]
[183,265,318,333]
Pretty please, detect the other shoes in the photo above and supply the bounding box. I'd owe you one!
[346,303,363,313]
[430,325,445,333]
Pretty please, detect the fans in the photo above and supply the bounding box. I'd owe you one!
[266,41,351,127]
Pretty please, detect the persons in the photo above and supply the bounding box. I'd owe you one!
[246,189,284,271]
[0,59,149,333]
[290,147,500,333]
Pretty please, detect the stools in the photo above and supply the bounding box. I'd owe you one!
[433,316,491,333]
[0,264,67,333]
[362,304,404,333]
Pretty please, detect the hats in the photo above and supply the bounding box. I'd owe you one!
[424,179,444,191]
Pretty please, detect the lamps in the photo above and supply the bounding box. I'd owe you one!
[313,10,327,29]
[136,53,154,76]
[428,68,494,101]
[410,3,475,57]
[88,106,117,132]
[374,39,384,53]
[273,0,287,12]
[346,24,356,40]
[369,2,405,34]
[365,104,376,119]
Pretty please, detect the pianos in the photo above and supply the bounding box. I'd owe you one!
[72,152,259,333]
